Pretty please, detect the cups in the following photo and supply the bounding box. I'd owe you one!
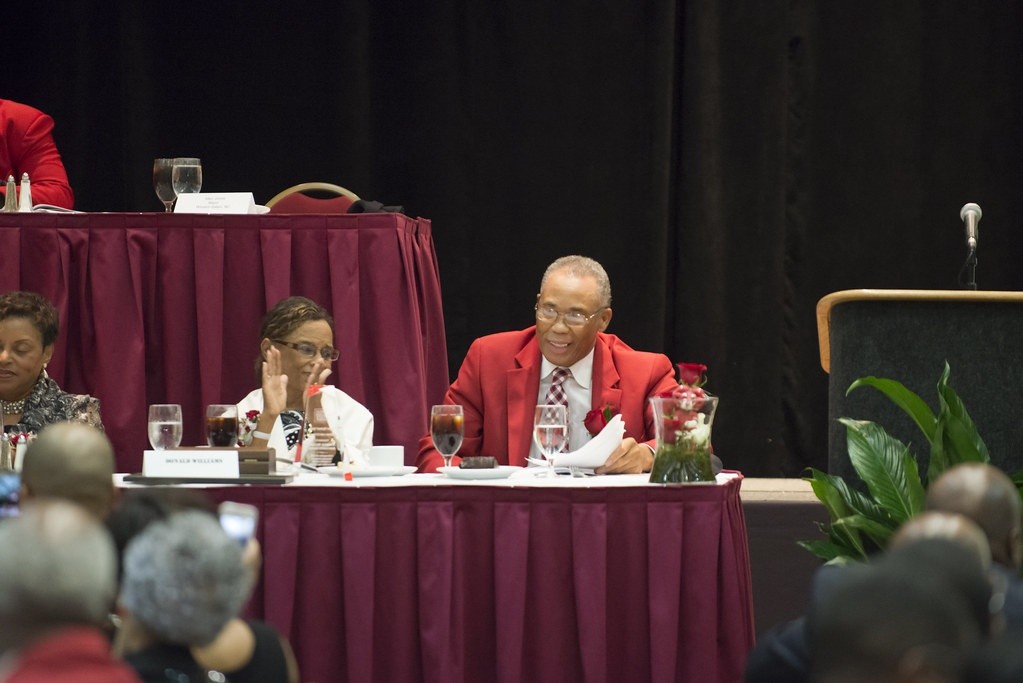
[369,445,404,466]
[148,403,184,451]
[205,405,239,448]
[170,158,202,197]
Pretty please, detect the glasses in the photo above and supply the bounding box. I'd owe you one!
[271,339,341,361]
[532,298,605,327]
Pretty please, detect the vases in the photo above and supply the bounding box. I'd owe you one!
[648,396,719,484]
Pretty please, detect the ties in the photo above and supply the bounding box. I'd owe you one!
[540,367,574,460]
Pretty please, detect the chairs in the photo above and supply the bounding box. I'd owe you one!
[265,182,360,214]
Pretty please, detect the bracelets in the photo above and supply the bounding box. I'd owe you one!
[253,430,272,441]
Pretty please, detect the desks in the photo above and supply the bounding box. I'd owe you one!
[114,465,759,683]
[0,207,450,468]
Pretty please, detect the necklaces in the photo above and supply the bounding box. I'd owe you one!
[0,399,27,416]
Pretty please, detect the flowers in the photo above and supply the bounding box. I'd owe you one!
[582,403,612,436]
[659,363,709,444]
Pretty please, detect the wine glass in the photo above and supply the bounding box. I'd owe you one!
[532,404,569,480]
[429,405,464,482]
[153,158,177,212]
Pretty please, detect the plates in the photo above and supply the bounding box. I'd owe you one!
[435,465,521,479]
[317,465,418,477]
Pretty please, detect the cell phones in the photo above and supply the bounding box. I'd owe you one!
[217,501,258,552]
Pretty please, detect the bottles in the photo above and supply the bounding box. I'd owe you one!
[17,172,34,212]
[0,175,17,213]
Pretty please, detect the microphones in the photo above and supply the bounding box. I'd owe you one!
[960,203,982,253]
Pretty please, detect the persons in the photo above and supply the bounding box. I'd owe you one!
[0,291,105,434]
[0,99,74,209]
[0,421,299,683]
[414,256,680,475]
[221,296,374,468]
[738,461,1023,683]
